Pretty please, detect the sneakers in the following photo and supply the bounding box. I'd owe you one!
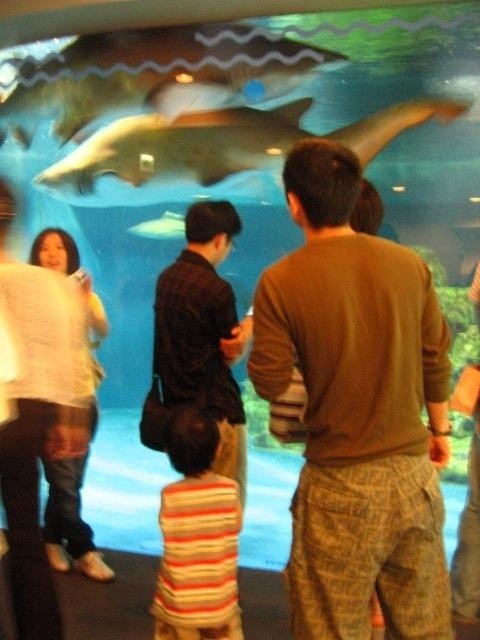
[75,546,115,581]
[44,541,70,571]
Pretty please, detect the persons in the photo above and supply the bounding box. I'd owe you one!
[147,405,247,640]
[266,176,382,445]
[25,225,116,582]
[246,138,460,639]
[450,361,480,640]
[149,198,250,515]
[0,175,87,640]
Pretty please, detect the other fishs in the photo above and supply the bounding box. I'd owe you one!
[125,210,187,241]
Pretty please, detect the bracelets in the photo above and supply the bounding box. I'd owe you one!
[425,417,454,437]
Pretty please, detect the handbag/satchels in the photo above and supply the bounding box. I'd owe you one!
[139,388,207,452]
